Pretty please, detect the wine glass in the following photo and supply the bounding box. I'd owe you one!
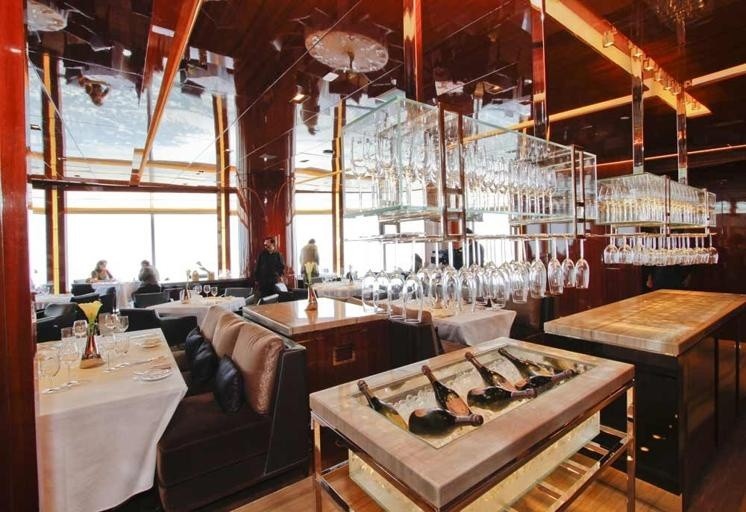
[345,103,594,225]
[178,284,218,304]
[37,312,132,395]
[358,236,593,324]
[595,182,707,224]
[321,270,357,288]
[602,234,720,268]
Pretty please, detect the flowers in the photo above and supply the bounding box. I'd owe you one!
[304,262,316,286]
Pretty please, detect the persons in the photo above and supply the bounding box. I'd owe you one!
[646,266,674,292]
[90,260,116,283]
[131,260,161,301]
[299,239,320,288]
[253,237,283,296]
[466,227,484,267]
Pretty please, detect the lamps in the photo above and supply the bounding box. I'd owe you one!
[24,1,73,37]
[300,24,389,77]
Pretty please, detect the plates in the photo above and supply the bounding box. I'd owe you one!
[138,337,171,382]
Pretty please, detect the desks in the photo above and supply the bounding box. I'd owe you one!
[240,297,390,394]
[541,287,746,511]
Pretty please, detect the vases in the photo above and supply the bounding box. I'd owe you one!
[306,285,317,304]
[81,324,101,359]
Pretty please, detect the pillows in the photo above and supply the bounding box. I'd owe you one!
[194,338,218,381]
[210,352,246,416]
[185,326,204,359]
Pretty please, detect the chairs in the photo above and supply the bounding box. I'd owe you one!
[32,279,311,349]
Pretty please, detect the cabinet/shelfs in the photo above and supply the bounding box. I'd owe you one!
[307,334,638,511]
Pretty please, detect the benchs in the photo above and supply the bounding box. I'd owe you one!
[153,305,313,512]
[389,315,470,367]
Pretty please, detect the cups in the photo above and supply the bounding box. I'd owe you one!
[40,286,50,296]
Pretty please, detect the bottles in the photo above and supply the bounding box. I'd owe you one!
[497,346,548,377]
[420,363,474,415]
[355,380,409,431]
[409,406,484,438]
[516,371,579,396]
[465,351,514,392]
[466,387,536,411]
